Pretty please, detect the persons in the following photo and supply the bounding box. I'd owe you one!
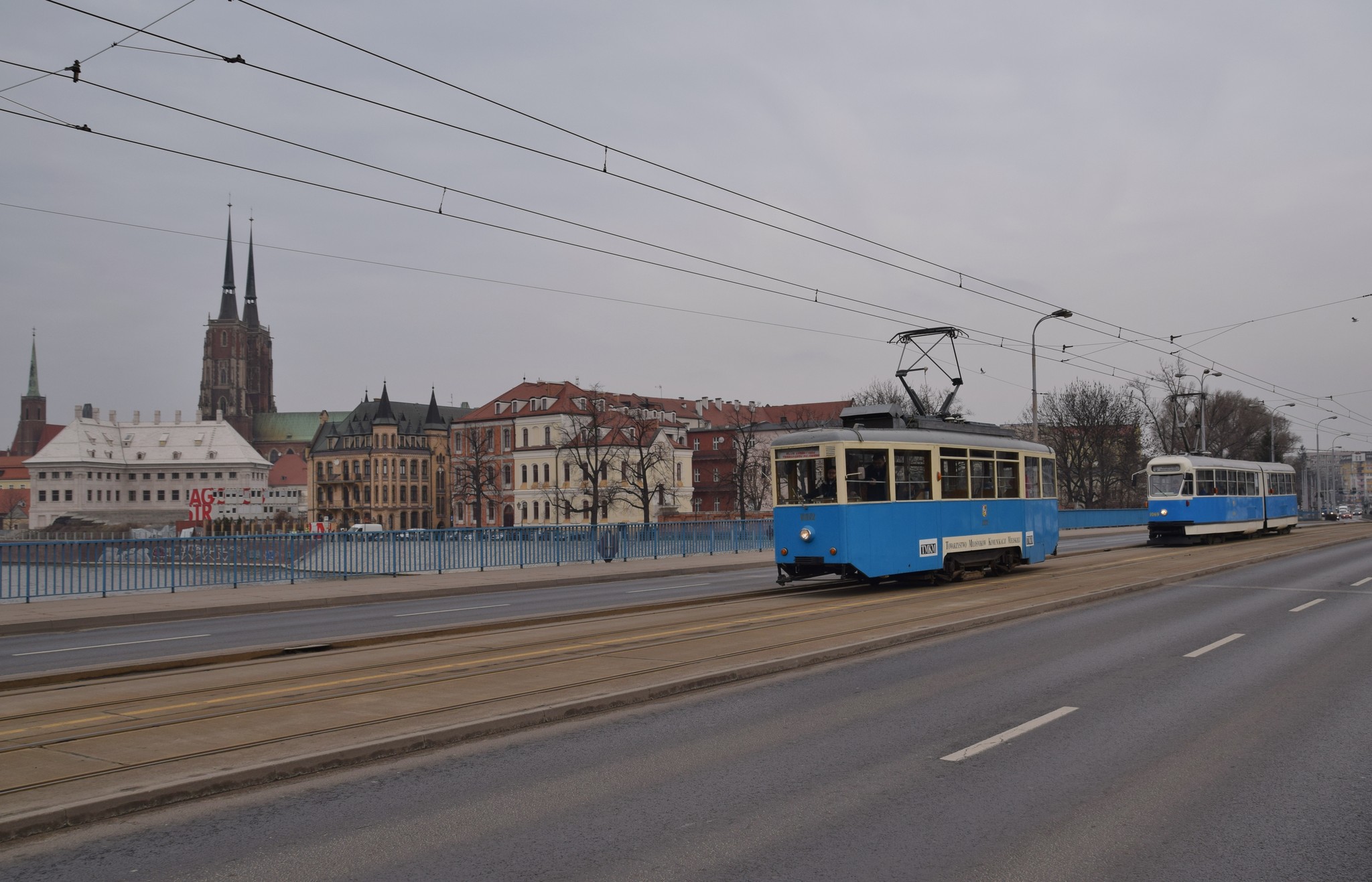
[906,478,920,495]
[799,466,856,504]
[1009,465,1032,498]
[896,481,916,500]
[1168,480,1184,495]
[1193,473,1299,499]
[865,454,898,502]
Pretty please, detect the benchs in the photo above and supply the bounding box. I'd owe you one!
[946,486,1019,499]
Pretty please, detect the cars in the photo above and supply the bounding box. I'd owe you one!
[398,526,587,542]
[1339,508,1363,519]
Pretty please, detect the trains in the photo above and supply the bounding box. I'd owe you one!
[770,313,1302,589]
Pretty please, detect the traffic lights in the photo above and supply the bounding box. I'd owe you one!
[1358,492,1361,494]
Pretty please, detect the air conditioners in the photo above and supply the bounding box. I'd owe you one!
[20,502,26,507]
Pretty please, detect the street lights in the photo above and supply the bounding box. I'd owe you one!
[1326,445,1342,517]
[1248,402,1296,462]
[1315,415,1338,520]
[516,502,524,526]
[1173,368,1224,456]
[1031,308,1073,443]
[1332,433,1350,514]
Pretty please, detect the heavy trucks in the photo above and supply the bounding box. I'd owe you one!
[1321,503,1333,517]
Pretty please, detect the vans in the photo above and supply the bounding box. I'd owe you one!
[347,524,383,540]
[1339,506,1348,513]
